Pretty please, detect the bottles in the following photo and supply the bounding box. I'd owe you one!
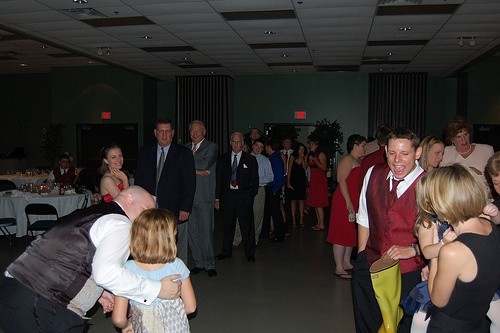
[59,180,64,195]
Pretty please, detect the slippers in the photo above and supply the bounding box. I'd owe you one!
[345,266,355,273]
[333,271,352,280]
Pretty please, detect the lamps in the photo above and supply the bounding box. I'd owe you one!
[97,47,103,56]
[459,35,464,47]
[105,47,112,56]
[469,36,477,48]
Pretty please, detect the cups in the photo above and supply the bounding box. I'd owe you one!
[67,189,71,194]
[20,182,48,193]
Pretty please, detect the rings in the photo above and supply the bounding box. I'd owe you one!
[107,304,111,307]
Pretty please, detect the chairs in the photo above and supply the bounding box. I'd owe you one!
[0,218,20,252]
[24,203,60,248]
[0,179,17,192]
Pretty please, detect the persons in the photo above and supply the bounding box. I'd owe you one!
[233,138,274,249]
[280,138,294,176]
[418,136,446,171]
[214,132,259,262]
[264,140,286,241]
[101,145,129,203]
[133,119,197,267]
[48,155,76,184]
[365,123,396,165]
[438,117,495,202]
[306,138,327,230]
[113,208,197,333]
[287,142,308,226]
[243,128,261,153]
[0,185,182,333]
[327,134,367,280]
[409,150,500,332]
[182,121,216,277]
[352,129,425,333]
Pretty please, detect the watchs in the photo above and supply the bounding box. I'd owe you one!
[411,244,418,255]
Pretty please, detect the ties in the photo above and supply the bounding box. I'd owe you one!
[284,151,288,171]
[156,147,164,184]
[389,178,404,207]
[192,144,196,155]
[232,155,237,187]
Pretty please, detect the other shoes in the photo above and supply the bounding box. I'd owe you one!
[207,269,217,277]
[268,224,320,241]
[190,267,201,274]
[247,256,256,263]
[214,255,233,260]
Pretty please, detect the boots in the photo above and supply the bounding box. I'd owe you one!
[369,251,404,333]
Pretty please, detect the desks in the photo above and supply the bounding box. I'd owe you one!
[0,187,92,238]
[0,169,55,190]
[0,153,28,174]
